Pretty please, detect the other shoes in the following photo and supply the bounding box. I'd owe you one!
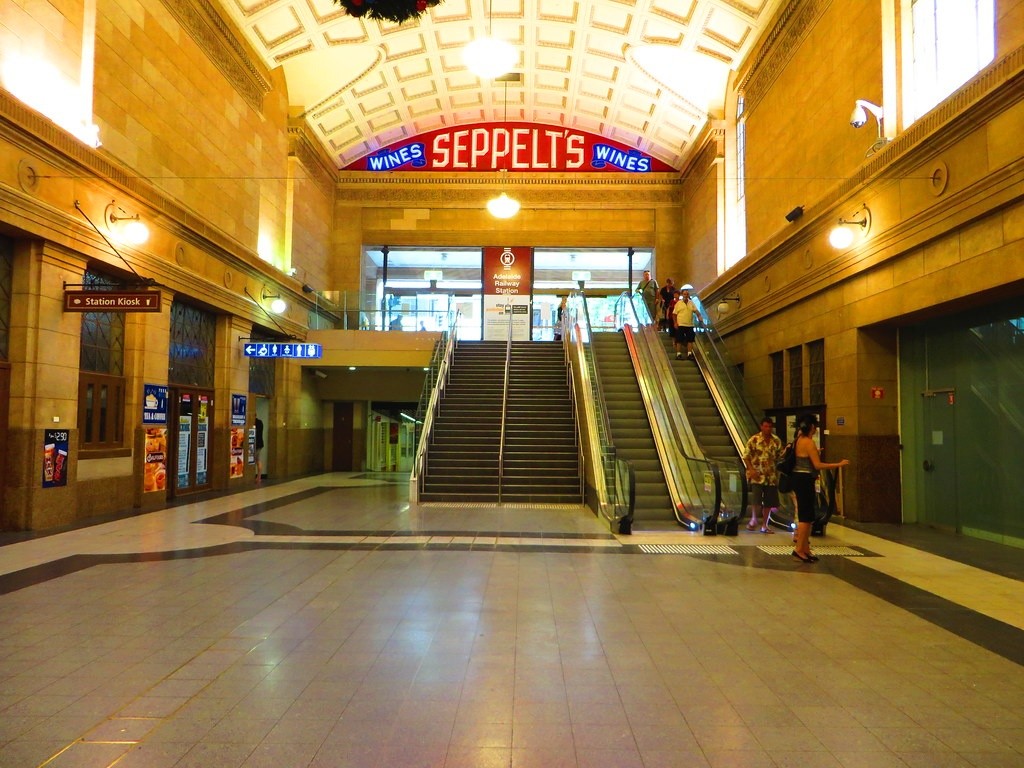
[807,554,818,560]
[792,551,814,563]
[687,354,694,359]
[675,354,686,359]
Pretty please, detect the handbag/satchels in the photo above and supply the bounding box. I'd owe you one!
[775,438,800,474]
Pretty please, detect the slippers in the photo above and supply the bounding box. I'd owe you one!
[747,523,755,530]
[761,527,774,533]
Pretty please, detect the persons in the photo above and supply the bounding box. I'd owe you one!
[256,411,264,483]
[389,315,404,331]
[420,320,427,331]
[780,413,824,545]
[741,416,782,534]
[634,270,707,362]
[791,420,851,565]
[553,295,568,340]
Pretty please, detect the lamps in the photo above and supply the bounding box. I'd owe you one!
[829,217,867,250]
[487,82,520,218]
[263,293,286,315]
[110,212,149,245]
[718,296,740,314]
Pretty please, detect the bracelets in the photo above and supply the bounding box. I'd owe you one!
[699,321,703,322]
[655,299,659,300]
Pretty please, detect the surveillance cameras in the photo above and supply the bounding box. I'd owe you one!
[850,106,866,128]
[315,370,328,379]
